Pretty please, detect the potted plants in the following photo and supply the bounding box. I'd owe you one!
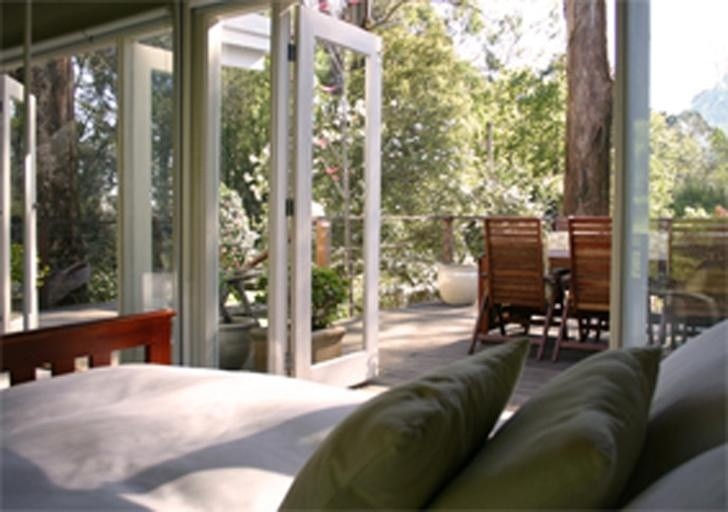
[248,265,352,376]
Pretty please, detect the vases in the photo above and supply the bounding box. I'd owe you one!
[214,314,260,371]
[435,262,482,307]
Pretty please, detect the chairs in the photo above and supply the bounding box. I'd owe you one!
[465,213,727,365]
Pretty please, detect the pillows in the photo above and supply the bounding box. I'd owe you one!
[617,441,728,512]
[422,344,664,511]
[276,333,529,511]
[637,315,728,478]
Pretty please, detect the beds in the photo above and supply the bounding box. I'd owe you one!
[1,306,728,511]
